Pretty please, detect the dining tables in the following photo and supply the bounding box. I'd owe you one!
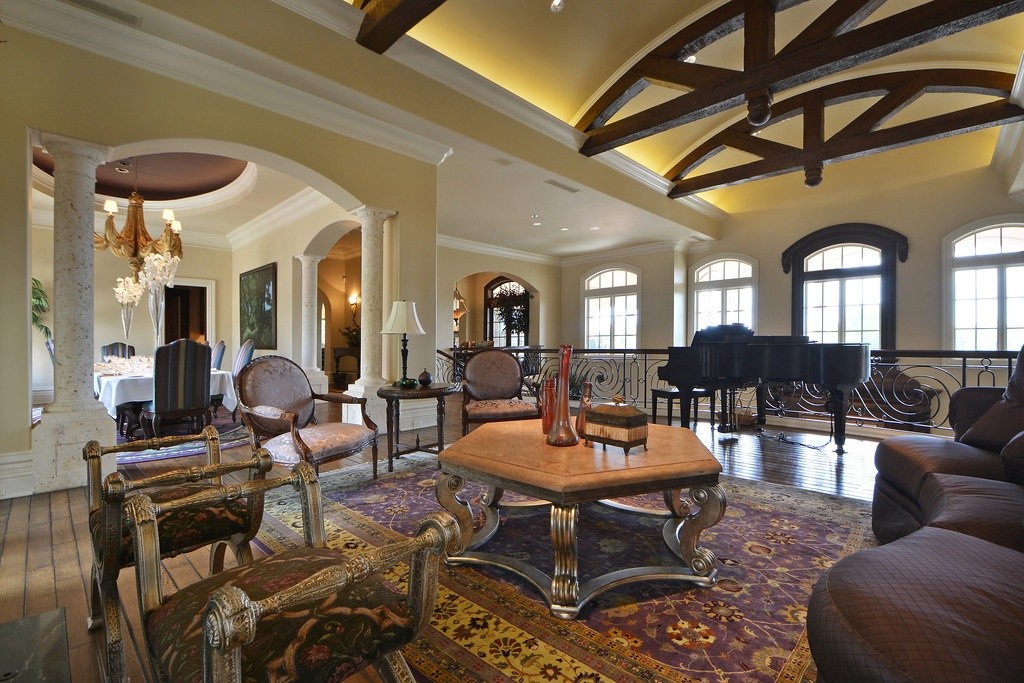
[94,355,237,442]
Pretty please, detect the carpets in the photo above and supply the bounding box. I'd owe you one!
[249,443,882,683]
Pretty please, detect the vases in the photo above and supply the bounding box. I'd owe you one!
[121,301,134,360]
[542,377,556,434]
[547,345,579,447]
[148,287,165,349]
[575,381,592,440]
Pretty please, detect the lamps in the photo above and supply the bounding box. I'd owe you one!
[92,154,182,284]
[380,299,426,387]
[453,281,468,326]
[349,295,357,307]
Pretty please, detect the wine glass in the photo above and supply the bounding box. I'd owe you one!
[104,354,154,376]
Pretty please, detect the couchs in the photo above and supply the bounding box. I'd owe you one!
[806,345,1024,683]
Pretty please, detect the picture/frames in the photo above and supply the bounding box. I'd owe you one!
[239,261,277,351]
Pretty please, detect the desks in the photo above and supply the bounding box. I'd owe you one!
[331,347,360,382]
[376,382,459,473]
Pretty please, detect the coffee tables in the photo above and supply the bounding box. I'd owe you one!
[434,415,727,620]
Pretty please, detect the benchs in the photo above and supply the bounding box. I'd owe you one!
[651,388,716,426]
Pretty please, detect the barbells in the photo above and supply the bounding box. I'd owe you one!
[657,324,869,454]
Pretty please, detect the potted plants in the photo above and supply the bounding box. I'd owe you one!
[338,318,361,347]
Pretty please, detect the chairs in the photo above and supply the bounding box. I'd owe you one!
[211,339,255,423]
[235,355,379,481]
[45,340,54,361]
[460,349,542,437]
[211,340,225,370]
[139,338,212,440]
[100,342,135,363]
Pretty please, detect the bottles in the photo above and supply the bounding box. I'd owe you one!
[418,367,433,386]
[544,378,557,435]
[576,382,593,438]
[546,345,579,446]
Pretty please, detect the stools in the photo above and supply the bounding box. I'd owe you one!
[82,425,272,683]
[124,460,461,683]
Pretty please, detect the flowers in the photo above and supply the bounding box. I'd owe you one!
[113,276,145,306]
[138,251,180,294]
